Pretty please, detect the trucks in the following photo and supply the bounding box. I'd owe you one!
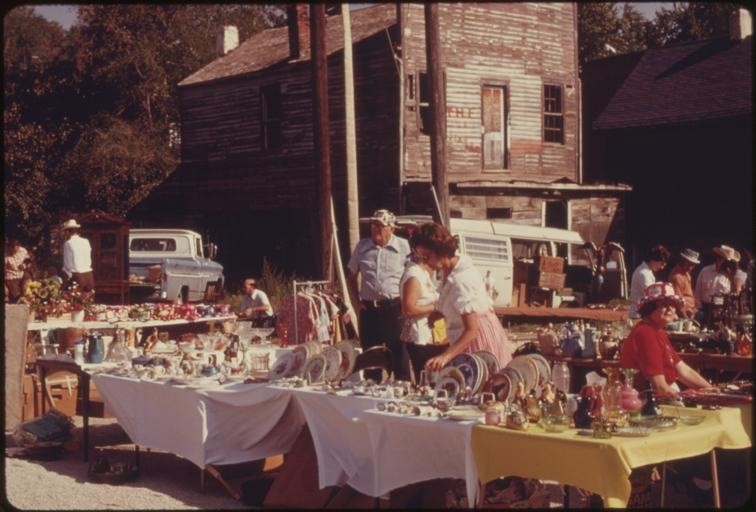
[358,212,628,310]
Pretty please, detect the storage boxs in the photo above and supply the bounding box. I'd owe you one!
[534,255,564,273]
[538,272,566,289]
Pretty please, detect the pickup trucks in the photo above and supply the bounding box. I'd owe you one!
[127,228,226,305]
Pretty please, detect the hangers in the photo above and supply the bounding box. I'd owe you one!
[298,279,335,296]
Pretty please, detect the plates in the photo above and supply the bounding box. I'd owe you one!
[449,408,484,420]
[268,341,355,381]
[434,351,551,404]
[681,415,705,425]
[632,417,677,427]
[619,428,649,436]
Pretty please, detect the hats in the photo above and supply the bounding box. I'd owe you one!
[359,209,395,227]
[713,245,741,262]
[60,218,81,232]
[680,249,701,265]
[633,281,684,311]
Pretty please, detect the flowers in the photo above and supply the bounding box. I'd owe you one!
[21,277,62,312]
[64,282,96,311]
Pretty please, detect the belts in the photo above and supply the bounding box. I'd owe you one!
[361,297,401,308]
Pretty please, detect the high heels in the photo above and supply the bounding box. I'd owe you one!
[684,478,726,504]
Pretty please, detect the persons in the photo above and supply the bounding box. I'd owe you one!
[5,239,30,304]
[617,282,711,408]
[695,244,748,325]
[400,222,512,380]
[667,248,701,320]
[57,219,95,303]
[237,279,276,328]
[347,210,412,384]
[629,246,672,325]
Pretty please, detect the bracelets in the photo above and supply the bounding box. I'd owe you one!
[253,307,256,313]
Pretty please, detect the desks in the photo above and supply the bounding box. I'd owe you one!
[550,347,702,391]
[701,353,752,377]
[92,365,478,512]
[36,348,140,462]
[27,312,238,347]
[472,399,756,512]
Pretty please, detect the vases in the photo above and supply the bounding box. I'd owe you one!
[42,308,64,322]
[72,310,85,322]
